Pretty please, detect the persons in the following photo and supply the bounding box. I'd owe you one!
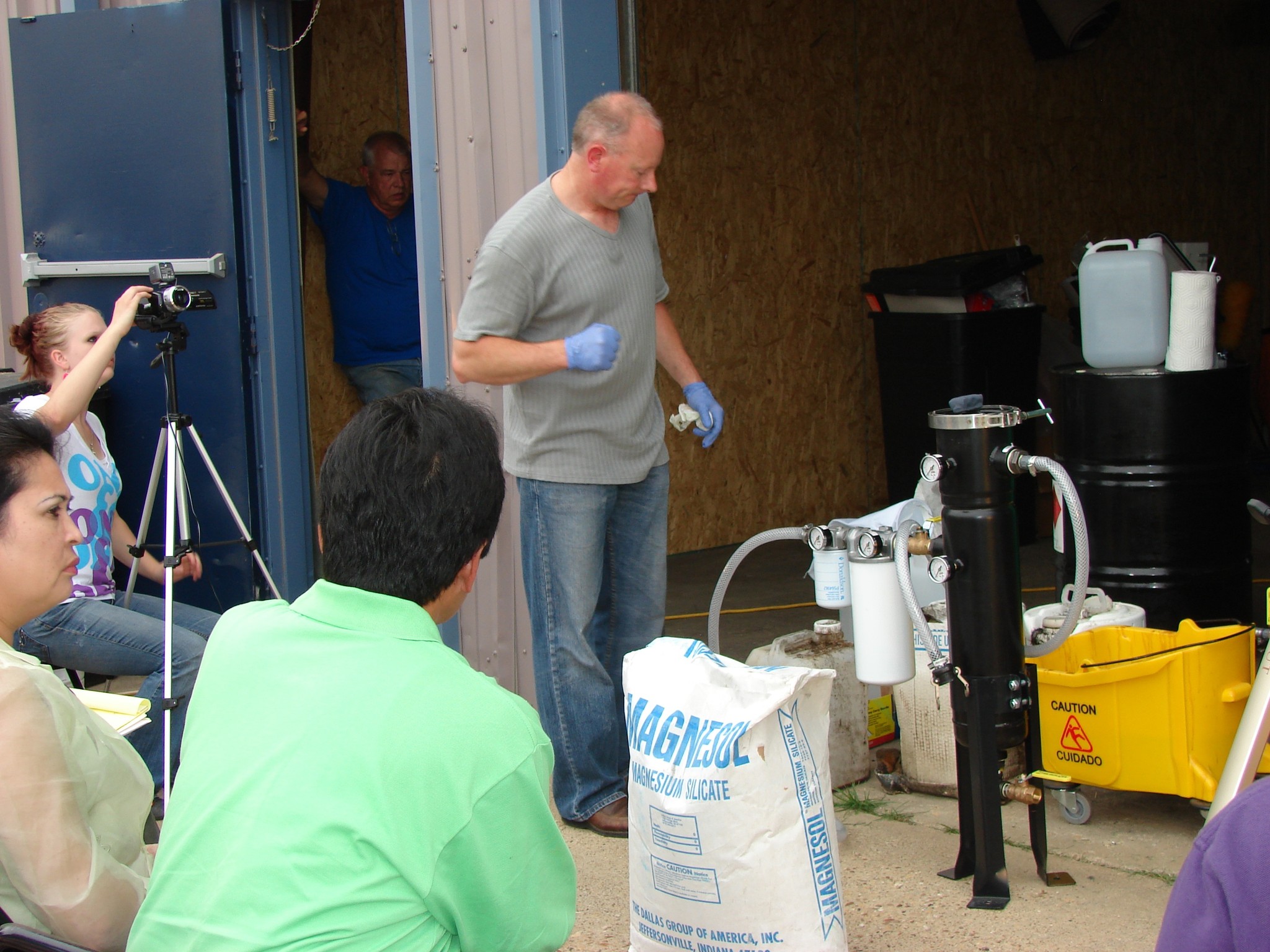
[1,408,160,952]
[10,285,221,820]
[454,92,725,838]
[296,107,422,406]
[125,386,578,952]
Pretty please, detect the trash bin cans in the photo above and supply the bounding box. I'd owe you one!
[866,243,1050,505]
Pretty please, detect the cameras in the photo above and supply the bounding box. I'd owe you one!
[136,262,216,322]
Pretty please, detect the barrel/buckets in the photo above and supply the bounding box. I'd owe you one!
[1077,237,1168,368]
[1043,354,1263,630]
[742,618,872,791]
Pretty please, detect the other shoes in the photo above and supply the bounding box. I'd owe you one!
[560,796,628,838]
[151,797,164,820]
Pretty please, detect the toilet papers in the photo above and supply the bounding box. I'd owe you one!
[1164,268,1218,373]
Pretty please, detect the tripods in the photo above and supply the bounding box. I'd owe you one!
[122,354,285,820]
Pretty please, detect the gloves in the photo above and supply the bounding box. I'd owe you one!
[683,382,726,448]
[563,322,621,372]
